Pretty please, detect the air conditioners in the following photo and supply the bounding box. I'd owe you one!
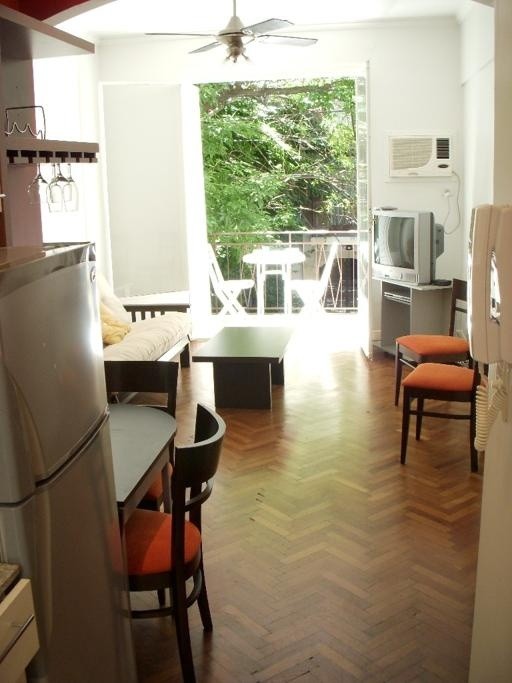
[388,134,453,179]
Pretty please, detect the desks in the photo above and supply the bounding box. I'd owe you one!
[243,247,307,322]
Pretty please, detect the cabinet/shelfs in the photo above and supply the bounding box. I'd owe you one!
[1,2,100,167]
[370,277,454,370]
[0,563,41,683]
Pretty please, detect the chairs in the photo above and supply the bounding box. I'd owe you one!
[401,316,489,473]
[104,361,179,608]
[290,242,338,320]
[395,278,472,405]
[208,244,256,322]
[123,403,226,682]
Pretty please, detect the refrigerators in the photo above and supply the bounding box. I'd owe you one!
[0,239,142,683]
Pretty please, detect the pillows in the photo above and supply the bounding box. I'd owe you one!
[101,293,133,324]
[100,302,125,344]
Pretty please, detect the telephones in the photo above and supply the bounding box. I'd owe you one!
[466,204,512,452]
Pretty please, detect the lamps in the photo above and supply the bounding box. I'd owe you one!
[223,36,250,64]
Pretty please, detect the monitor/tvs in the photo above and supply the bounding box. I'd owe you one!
[372,210,445,286]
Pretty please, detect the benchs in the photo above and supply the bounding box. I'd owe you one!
[99,290,191,407]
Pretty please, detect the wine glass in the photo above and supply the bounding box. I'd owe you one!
[25,159,80,216]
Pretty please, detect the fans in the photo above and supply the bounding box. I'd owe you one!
[144,0,318,53]
